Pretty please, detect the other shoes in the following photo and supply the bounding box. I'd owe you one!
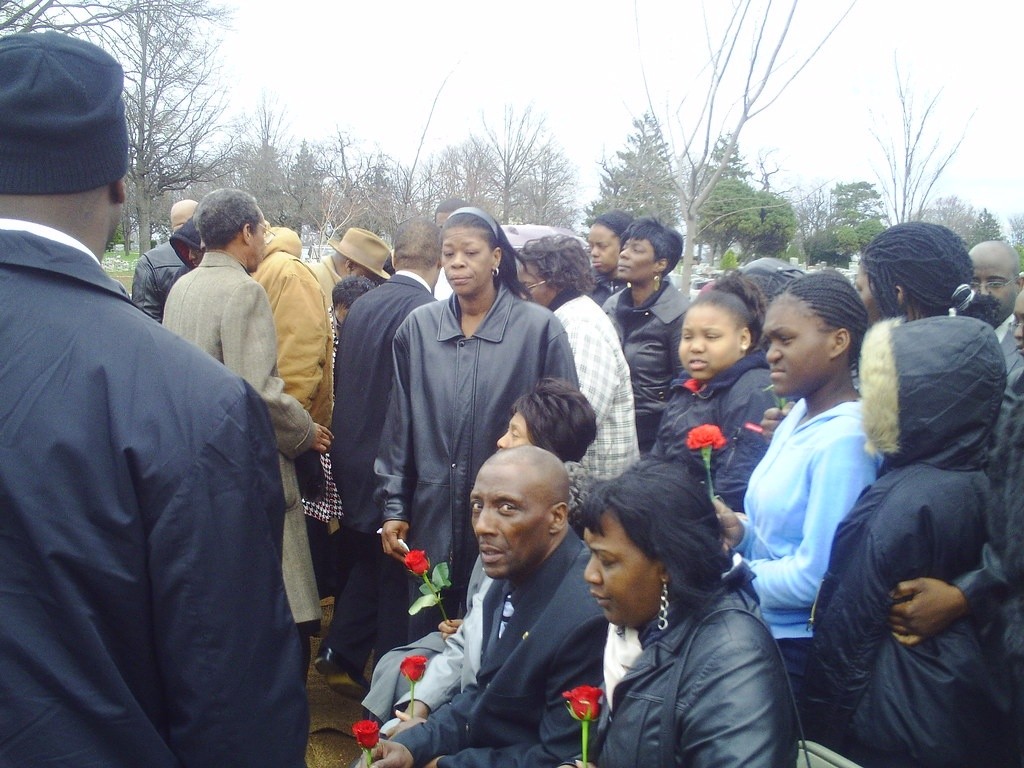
[314,649,369,700]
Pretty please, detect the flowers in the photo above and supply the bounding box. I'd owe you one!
[351,719,380,765]
[561,686,604,768]
[686,425,725,499]
[399,656,427,717]
[402,548,450,620]
[684,378,697,392]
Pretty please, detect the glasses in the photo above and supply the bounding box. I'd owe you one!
[969,274,1020,294]
[239,219,276,245]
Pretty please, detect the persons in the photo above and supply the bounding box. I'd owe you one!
[138,188,335,682]
[314,205,691,689]
[0,30,310,768]
[351,367,798,768]
[654,222,1023,766]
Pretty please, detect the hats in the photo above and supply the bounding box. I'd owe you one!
[0,32,129,193]
[328,227,392,281]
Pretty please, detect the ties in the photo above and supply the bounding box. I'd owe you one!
[497,594,516,640]
